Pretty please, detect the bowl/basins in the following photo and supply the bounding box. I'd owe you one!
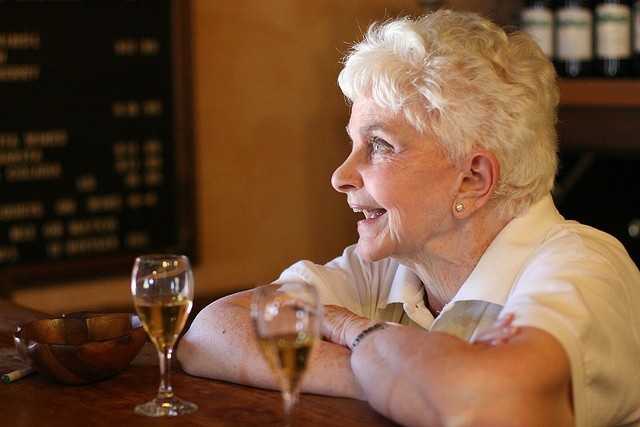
[14,312,147,386]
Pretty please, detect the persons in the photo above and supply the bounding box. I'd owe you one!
[175,9,640,427]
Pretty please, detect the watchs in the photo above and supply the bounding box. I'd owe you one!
[350,322,400,351]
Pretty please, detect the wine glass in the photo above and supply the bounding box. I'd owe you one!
[130,254,195,416]
[249,279,319,426]
